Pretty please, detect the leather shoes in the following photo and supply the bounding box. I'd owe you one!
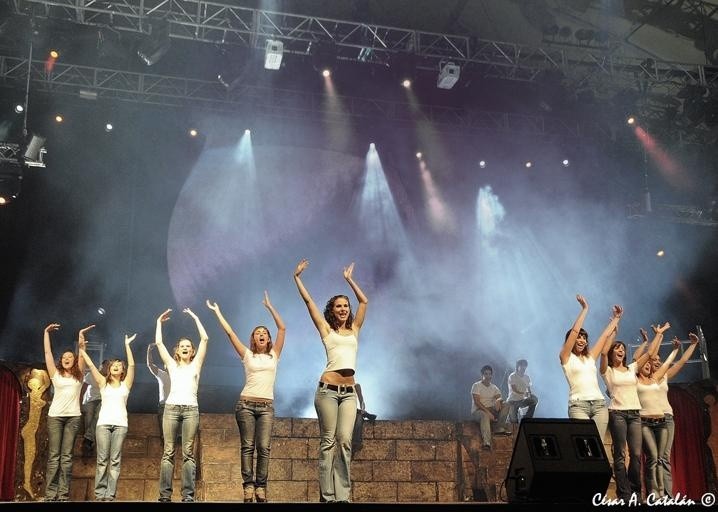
[495,431,512,436]
[482,444,490,449]
[244,492,267,502]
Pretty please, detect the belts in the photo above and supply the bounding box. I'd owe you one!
[641,417,665,423]
[319,382,353,392]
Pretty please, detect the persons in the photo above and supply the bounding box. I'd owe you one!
[506,360,538,424]
[471,366,512,451]
[206,290,286,503]
[559,294,699,506]
[39,306,209,504]
[350,383,365,459]
[701,390,718,479]
[18,368,51,500]
[293,258,368,503]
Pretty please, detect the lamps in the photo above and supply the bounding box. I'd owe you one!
[25,133,49,168]
[49,24,461,91]
